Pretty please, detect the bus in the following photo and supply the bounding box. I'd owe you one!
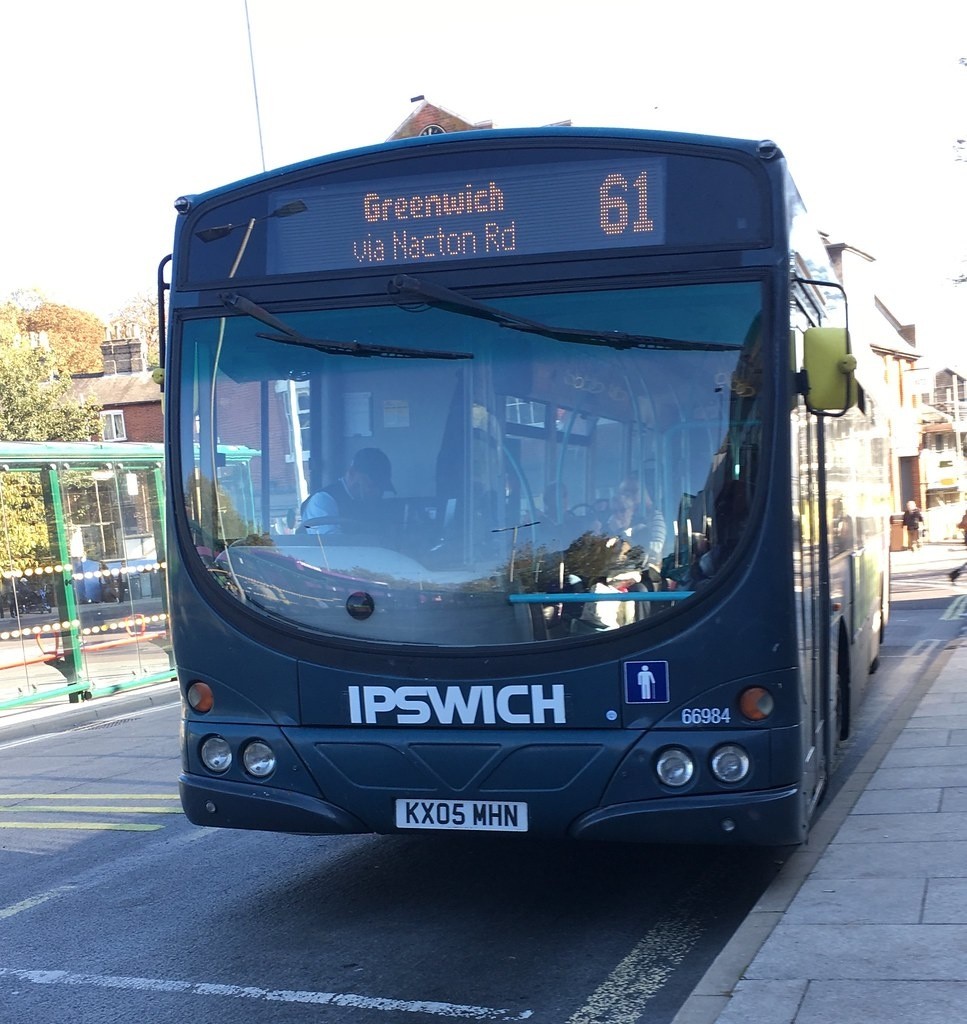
[156,121,896,877]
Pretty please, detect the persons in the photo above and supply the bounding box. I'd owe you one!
[520,444,758,632]
[100,560,128,605]
[17,578,37,608]
[901,499,924,552]
[297,449,392,539]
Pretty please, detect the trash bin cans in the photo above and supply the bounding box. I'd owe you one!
[890,512,906,552]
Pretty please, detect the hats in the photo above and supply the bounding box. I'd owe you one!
[353,448,397,495]
